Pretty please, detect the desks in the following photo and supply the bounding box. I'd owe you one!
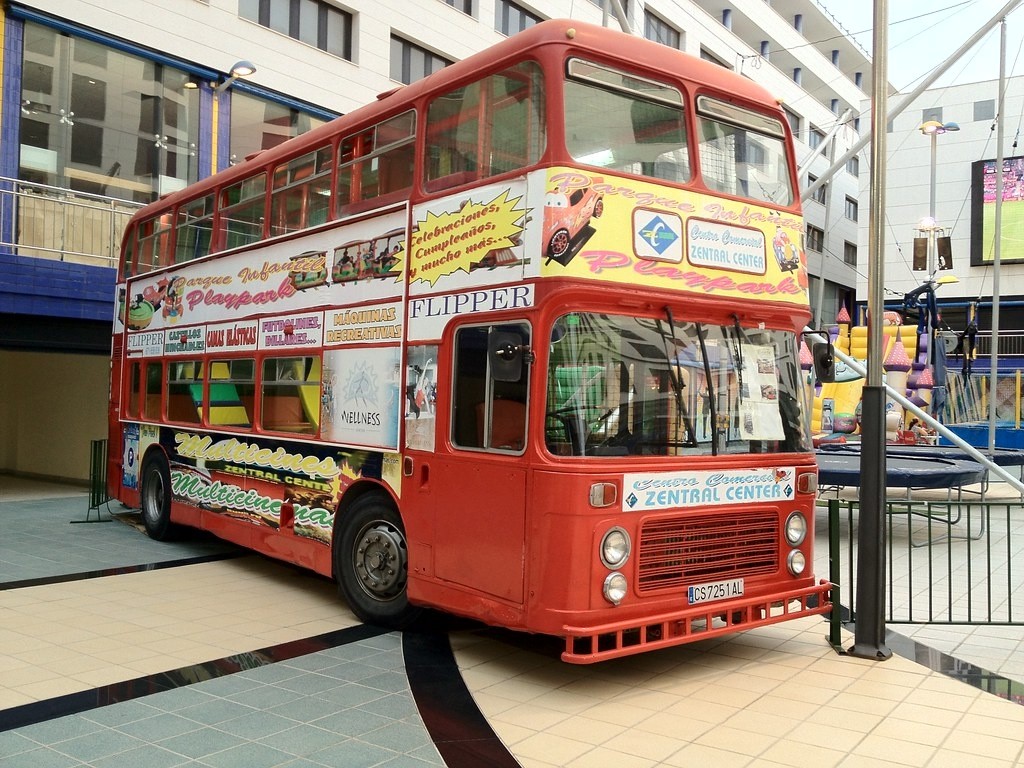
[921,436,942,445]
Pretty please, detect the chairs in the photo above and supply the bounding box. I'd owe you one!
[476,401,527,451]
[128,356,322,436]
[903,431,917,445]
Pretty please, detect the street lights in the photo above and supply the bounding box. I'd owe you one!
[184,62,257,176]
[920,120,960,415]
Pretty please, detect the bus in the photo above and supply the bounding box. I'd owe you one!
[110,20,831,665]
